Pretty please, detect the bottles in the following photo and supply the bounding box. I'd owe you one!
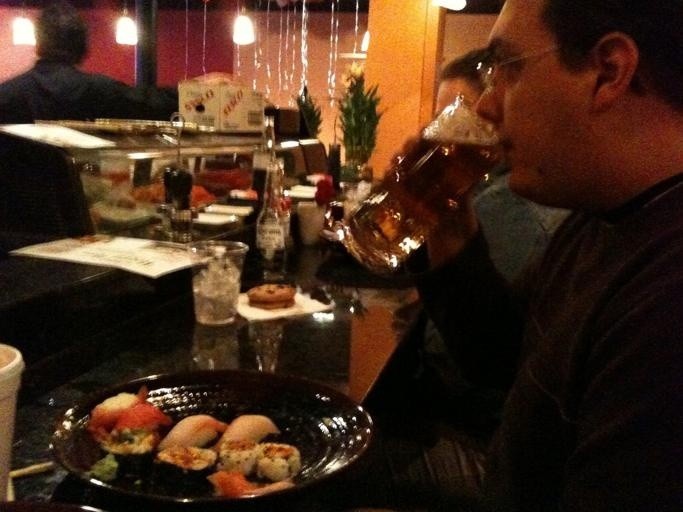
[252,160,298,311]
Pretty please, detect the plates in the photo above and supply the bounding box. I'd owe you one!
[46,365,378,506]
[191,203,254,232]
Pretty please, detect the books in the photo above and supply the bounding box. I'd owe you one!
[8,231,226,280]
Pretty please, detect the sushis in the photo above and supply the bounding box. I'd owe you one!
[257,443,302,482]
[220,438,258,478]
[99,428,158,478]
[151,447,218,496]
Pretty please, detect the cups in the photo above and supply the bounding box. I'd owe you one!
[185,240,251,329]
[295,200,326,248]
[0,344,26,502]
[327,88,514,280]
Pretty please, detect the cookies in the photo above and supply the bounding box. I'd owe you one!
[247,283,295,308]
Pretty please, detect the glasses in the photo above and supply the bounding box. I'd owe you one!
[474,45,560,86]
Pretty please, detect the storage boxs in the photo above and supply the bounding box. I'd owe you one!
[176,82,268,133]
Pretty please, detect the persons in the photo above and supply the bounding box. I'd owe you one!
[0,3,280,124]
